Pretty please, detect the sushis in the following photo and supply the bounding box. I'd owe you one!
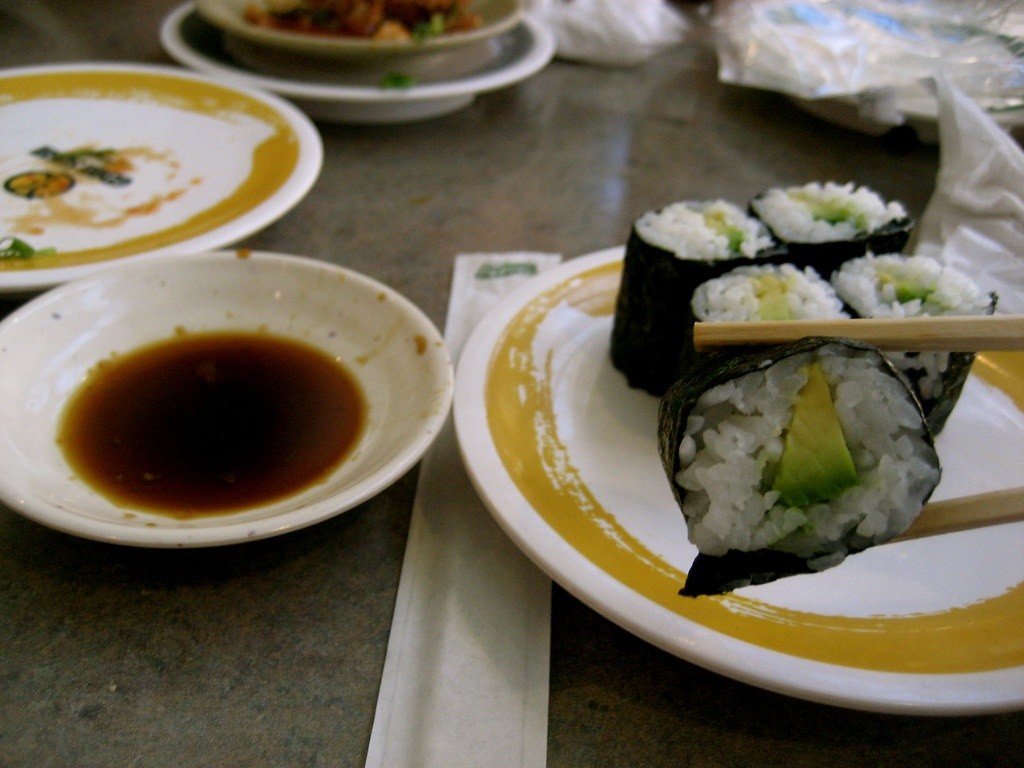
[611,180,1001,603]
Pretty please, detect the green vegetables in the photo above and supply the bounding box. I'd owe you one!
[380,12,448,90]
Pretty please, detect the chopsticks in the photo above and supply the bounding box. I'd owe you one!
[691,317,1023,546]
[366,251,549,768]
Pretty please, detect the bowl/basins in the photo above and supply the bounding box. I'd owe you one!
[199,0,533,85]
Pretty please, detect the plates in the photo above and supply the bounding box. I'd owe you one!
[0,250,452,549]
[158,0,551,119]
[0,64,323,293]
[454,243,1020,717]
